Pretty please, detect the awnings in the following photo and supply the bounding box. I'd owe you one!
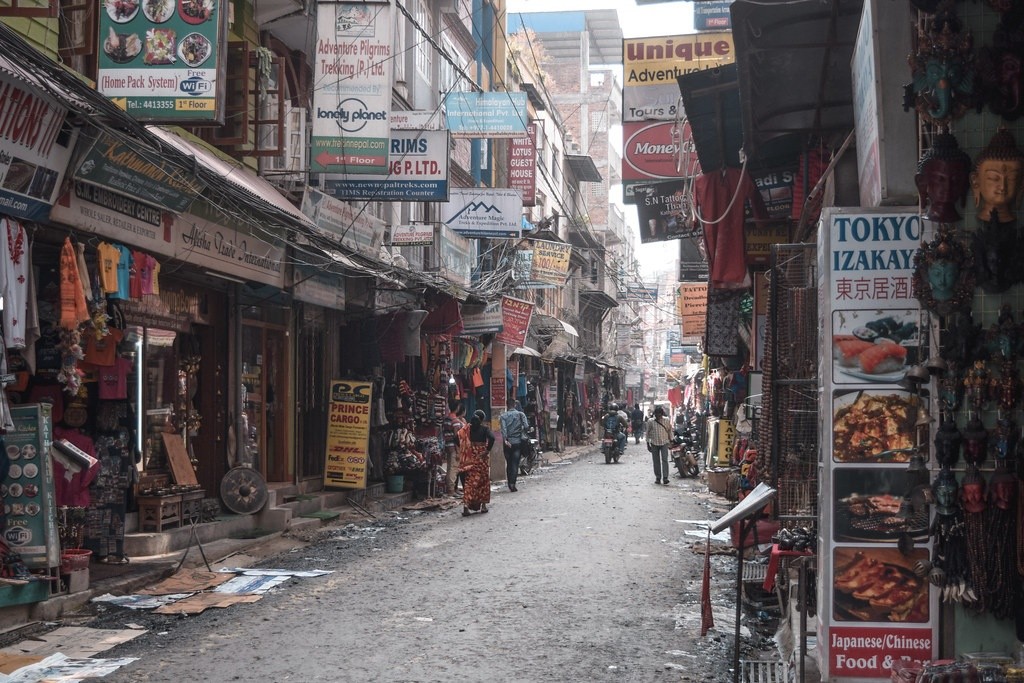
[579,290,618,323]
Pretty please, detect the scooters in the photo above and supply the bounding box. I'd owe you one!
[520,427,539,475]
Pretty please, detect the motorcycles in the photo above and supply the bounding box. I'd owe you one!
[601,418,630,465]
[669,436,699,478]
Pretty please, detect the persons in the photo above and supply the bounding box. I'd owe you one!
[600,403,632,455]
[514,400,534,474]
[631,403,644,444]
[455,410,495,516]
[500,398,529,491]
[646,407,674,484]
[443,399,464,494]
[455,403,468,493]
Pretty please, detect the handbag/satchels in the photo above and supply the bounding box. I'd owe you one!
[521,435,533,458]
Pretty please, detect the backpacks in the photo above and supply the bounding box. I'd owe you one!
[606,411,618,432]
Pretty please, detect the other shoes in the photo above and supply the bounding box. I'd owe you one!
[481,508,489,512]
[462,509,470,515]
[656,479,660,483]
[664,479,669,484]
[509,483,518,492]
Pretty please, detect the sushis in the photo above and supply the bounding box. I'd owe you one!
[832,315,922,375]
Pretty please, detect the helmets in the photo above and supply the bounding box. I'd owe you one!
[609,403,619,412]
